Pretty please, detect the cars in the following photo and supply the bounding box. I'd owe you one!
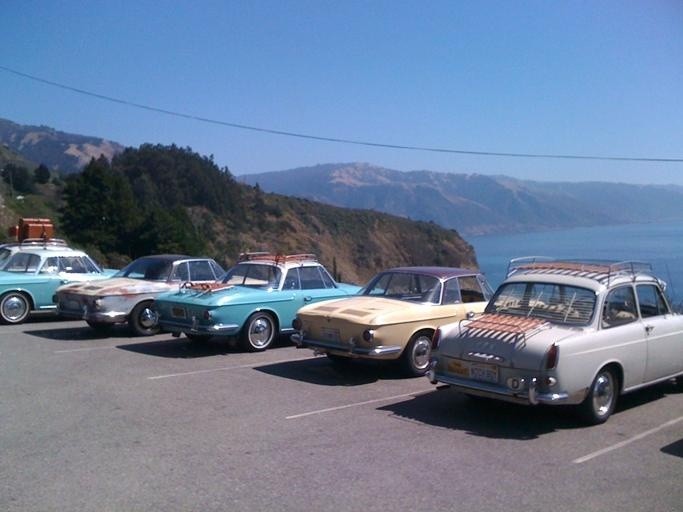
[0,238,143,325]
[290,266,546,377]
[150,252,393,352]
[53,254,269,336]
[424,255,683,423]
